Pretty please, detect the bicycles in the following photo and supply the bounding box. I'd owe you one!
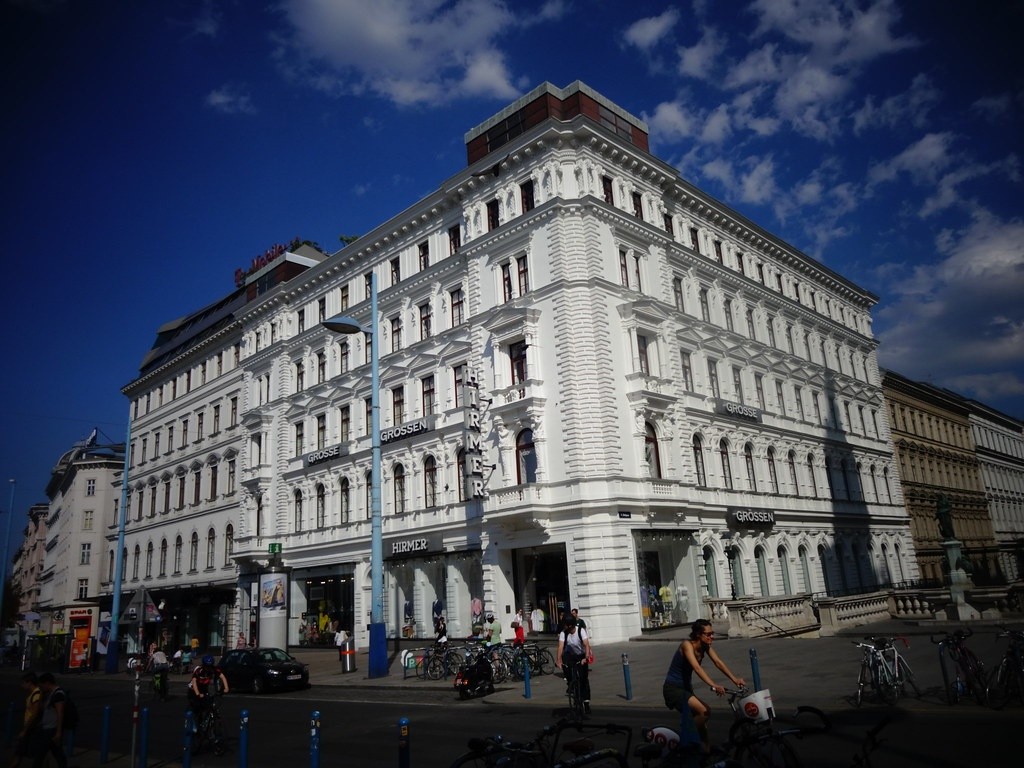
[849,631,924,707]
[412,633,556,699]
[182,687,229,757]
[144,669,170,699]
[930,622,1024,711]
[445,685,833,768]
[845,715,892,768]
[554,657,592,733]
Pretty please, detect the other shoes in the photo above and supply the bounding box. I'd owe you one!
[584,702,591,713]
[566,688,573,693]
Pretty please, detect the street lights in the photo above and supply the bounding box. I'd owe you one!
[317,271,392,680]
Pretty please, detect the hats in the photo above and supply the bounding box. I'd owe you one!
[566,616,576,623]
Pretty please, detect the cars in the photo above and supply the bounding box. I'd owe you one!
[213,647,310,696]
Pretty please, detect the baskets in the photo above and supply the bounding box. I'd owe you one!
[738,689,776,726]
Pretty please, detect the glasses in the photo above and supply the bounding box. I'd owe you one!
[702,632,714,637]
[567,623,574,626]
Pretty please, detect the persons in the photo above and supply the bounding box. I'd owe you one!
[639,579,691,628]
[555,609,591,714]
[333,625,349,661]
[301,621,319,646]
[31,673,67,768]
[8,673,40,768]
[190,635,199,651]
[514,609,525,647]
[171,647,192,675]
[433,617,447,649]
[662,619,746,753]
[248,634,256,647]
[144,648,168,694]
[187,655,229,733]
[486,614,502,669]
[235,632,248,649]
[127,640,158,675]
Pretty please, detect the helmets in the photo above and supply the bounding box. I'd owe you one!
[202,655,214,667]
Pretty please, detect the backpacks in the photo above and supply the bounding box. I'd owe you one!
[565,627,593,664]
[51,689,80,729]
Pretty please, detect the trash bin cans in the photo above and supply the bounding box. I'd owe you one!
[341,637,355,673]
